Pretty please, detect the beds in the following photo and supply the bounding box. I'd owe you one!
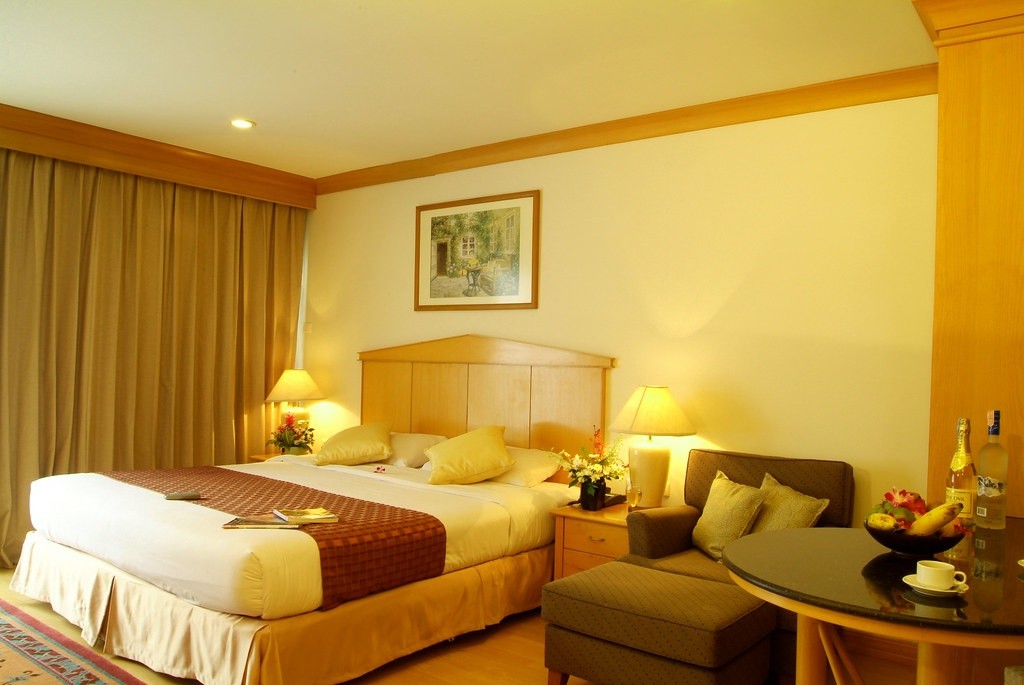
[11,336,618,685]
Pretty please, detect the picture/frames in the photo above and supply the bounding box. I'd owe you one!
[414,189,542,313]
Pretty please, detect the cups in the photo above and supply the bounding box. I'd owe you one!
[916,560,966,590]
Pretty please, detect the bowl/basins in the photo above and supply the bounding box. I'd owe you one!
[864,518,965,559]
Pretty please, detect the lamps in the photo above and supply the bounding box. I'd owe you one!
[267,369,327,430]
[607,384,698,509]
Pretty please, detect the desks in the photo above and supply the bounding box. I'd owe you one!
[720,527,1024,685]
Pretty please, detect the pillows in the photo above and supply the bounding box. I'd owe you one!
[420,446,563,486]
[383,431,450,468]
[424,427,515,485]
[313,422,394,466]
[749,472,830,536]
[689,470,768,564]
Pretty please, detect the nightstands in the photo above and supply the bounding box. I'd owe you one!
[554,504,651,582]
[250,450,316,463]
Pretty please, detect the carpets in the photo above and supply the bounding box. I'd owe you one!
[0,594,145,685]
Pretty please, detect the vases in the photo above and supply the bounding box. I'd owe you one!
[283,443,308,454]
[578,477,607,511]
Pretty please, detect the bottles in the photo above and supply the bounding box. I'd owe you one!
[975,410,1008,529]
[944,417,978,526]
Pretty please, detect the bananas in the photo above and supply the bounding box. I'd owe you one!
[868,502,964,537]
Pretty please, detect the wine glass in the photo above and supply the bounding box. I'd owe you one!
[626,481,643,512]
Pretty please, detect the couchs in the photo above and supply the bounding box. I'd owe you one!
[618,448,856,685]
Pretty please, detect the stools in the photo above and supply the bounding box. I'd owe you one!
[541,561,777,685]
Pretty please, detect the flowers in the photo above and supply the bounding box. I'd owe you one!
[557,425,633,482]
[267,415,320,454]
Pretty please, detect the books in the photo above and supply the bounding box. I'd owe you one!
[274,508,339,523]
[223,517,302,529]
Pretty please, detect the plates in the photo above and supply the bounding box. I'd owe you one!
[1017,560,1024,566]
[902,574,970,597]
